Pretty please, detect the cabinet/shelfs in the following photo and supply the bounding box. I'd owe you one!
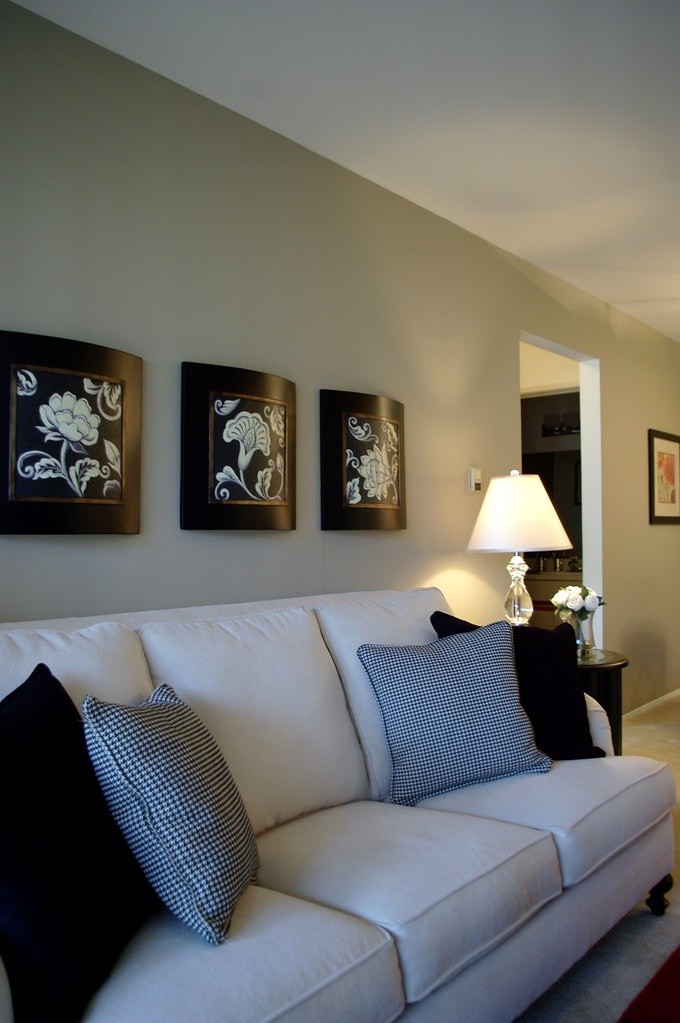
[524,581,584,627]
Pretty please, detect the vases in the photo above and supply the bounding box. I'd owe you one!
[570,611,596,665]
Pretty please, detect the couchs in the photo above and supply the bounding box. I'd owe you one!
[1,588,677,1023]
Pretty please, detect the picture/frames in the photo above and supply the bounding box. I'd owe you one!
[318,389,407,532]
[649,429,680,524]
[180,361,297,532]
[0,330,144,535]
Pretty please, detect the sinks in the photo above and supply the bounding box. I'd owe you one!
[525,571,581,604]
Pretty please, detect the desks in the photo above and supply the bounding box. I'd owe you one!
[570,649,629,756]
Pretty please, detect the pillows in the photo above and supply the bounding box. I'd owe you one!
[431,610,607,761]
[357,621,554,807]
[82,684,263,946]
[0,663,171,1022]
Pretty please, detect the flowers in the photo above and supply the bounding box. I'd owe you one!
[549,584,606,624]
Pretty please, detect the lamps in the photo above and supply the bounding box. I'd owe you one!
[465,470,574,626]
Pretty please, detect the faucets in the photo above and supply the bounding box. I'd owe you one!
[550,558,562,571]
